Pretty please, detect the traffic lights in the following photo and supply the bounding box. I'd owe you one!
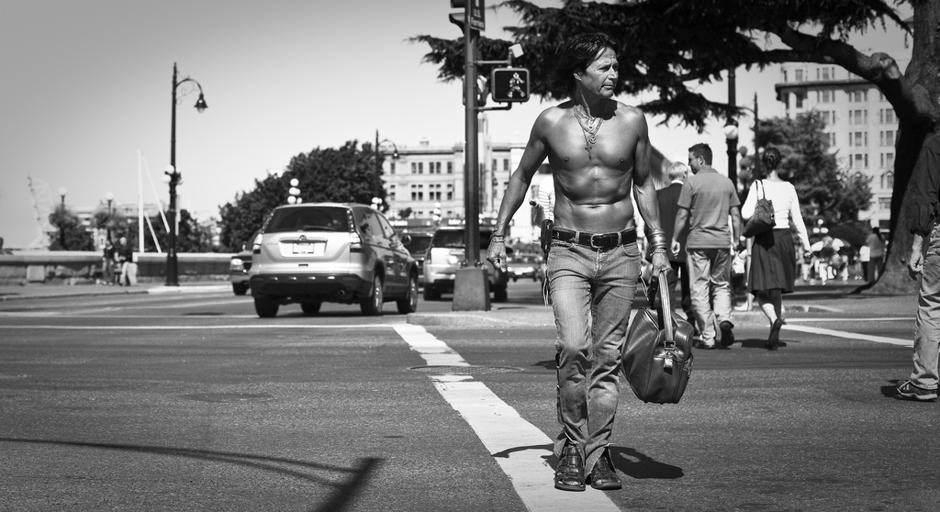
[490,68,530,103]
[449,0,469,33]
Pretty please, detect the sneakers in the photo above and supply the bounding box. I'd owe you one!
[693,318,787,353]
[891,375,939,404]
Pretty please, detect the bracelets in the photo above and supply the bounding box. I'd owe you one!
[489,229,511,244]
[648,230,669,253]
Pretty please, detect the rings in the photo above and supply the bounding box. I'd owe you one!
[492,260,500,264]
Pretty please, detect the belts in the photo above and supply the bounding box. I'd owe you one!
[548,223,639,253]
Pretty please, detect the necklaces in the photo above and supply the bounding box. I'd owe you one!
[570,101,613,161]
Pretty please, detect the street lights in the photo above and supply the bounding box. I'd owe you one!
[165,60,208,287]
[103,192,116,245]
[57,188,68,248]
[287,175,306,205]
[720,112,739,186]
[371,131,399,211]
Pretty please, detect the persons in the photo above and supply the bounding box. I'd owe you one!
[884,126,939,402]
[796,225,885,290]
[642,159,698,341]
[115,234,141,287]
[484,29,673,493]
[101,237,115,285]
[739,144,813,348]
[668,143,745,351]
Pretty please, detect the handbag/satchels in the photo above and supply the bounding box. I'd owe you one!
[740,200,777,240]
[626,305,688,404]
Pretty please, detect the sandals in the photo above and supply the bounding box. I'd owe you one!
[588,446,625,492]
[554,442,586,491]
